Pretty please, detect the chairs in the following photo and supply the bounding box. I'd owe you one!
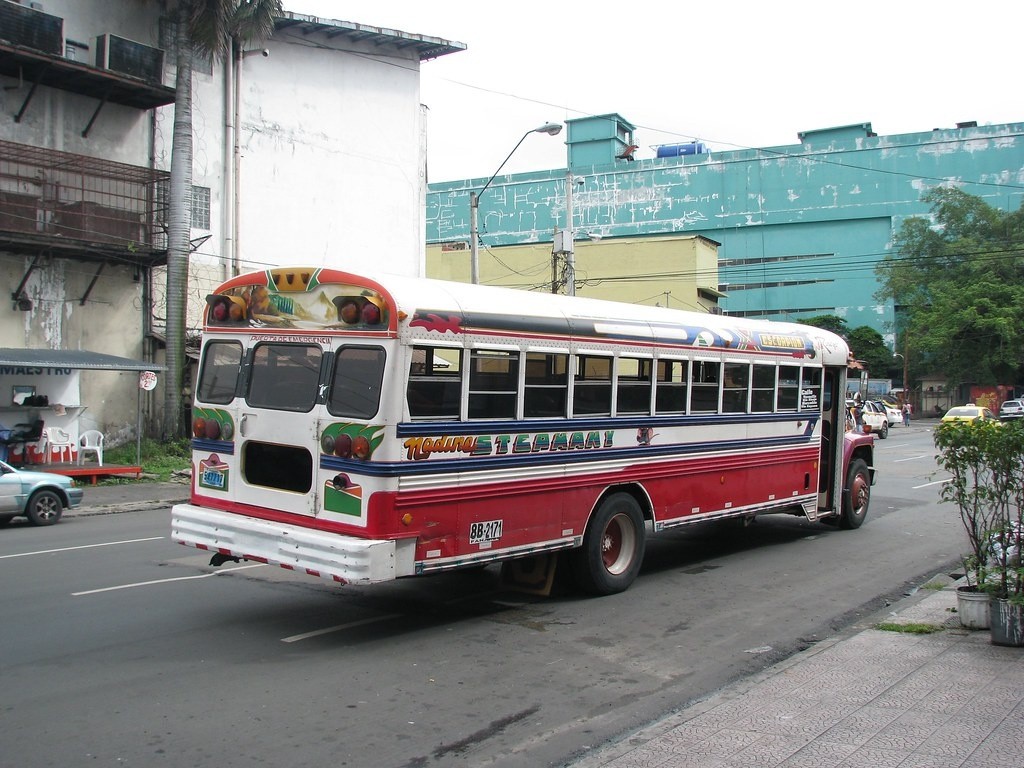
[1,420,45,468]
[77,429,104,466]
[43,427,74,463]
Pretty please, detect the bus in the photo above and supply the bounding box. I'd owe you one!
[170,264,879,599]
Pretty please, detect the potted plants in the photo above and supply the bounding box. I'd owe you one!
[931,416,1024,646]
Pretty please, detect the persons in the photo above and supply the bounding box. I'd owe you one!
[902,402,911,426]
[855,407,865,433]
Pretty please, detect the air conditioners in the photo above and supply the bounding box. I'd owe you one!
[0,0,66,58]
[97,31,166,85]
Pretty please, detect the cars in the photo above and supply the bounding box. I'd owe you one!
[939,403,1003,438]
[999,395,1024,422]
[824,387,909,439]
[0,461,84,527]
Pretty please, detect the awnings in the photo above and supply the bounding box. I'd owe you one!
[0,347,168,467]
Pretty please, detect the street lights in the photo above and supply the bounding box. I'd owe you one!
[893,352,907,404]
[470,122,563,283]
